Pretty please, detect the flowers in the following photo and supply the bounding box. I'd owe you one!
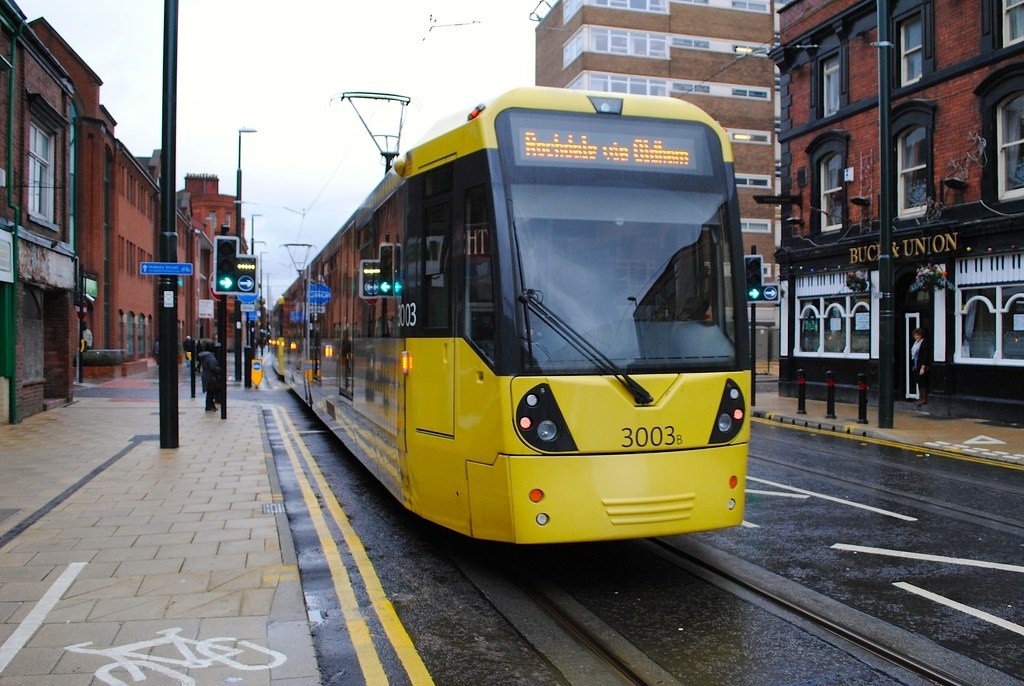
[909,263,957,292]
[848,270,866,291]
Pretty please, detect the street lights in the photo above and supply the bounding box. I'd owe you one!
[235,128,257,382]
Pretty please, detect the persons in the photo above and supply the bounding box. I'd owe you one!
[195,340,202,373]
[911,329,927,405]
[153,339,160,366]
[182,336,192,367]
[201,343,222,412]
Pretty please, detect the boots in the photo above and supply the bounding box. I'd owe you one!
[914,389,927,405]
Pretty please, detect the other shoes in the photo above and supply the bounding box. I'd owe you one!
[205,407,217,413]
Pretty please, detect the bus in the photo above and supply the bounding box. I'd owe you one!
[266,84,752,544]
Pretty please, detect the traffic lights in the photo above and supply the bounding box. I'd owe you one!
[742,254,764,303]
[212,234,240,295]
